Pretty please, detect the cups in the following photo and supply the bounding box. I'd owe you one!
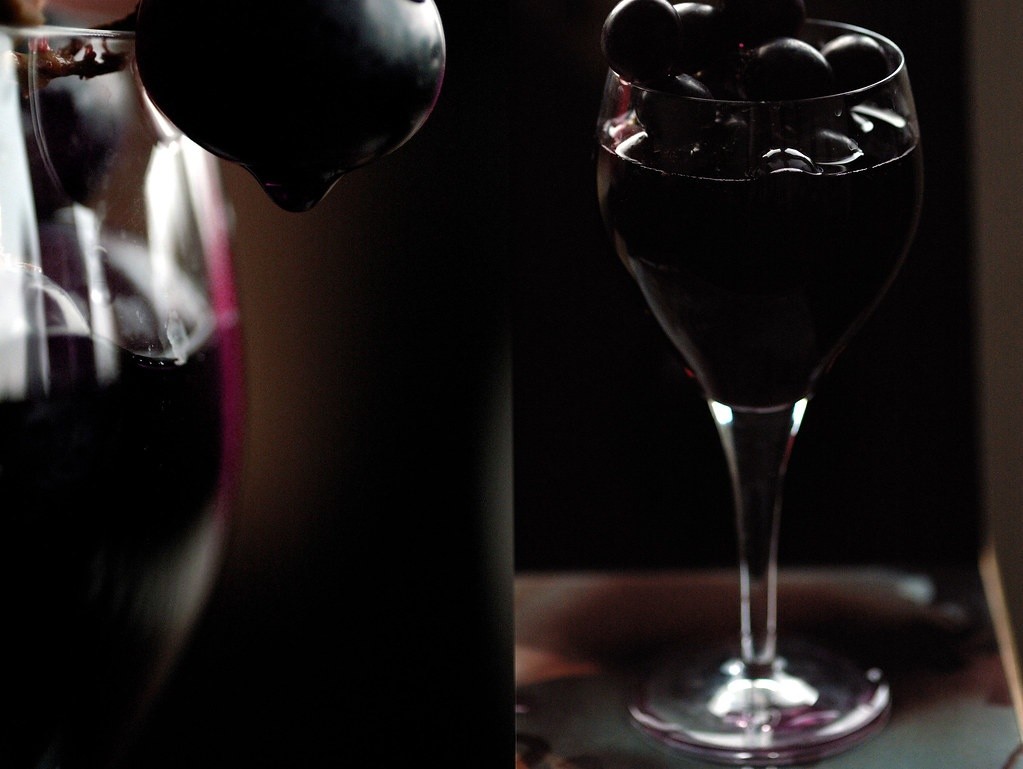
[0,21,253,769]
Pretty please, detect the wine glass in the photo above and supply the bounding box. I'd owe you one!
[594,15,925,768]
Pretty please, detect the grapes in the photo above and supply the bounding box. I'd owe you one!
[600,0,892,150]
